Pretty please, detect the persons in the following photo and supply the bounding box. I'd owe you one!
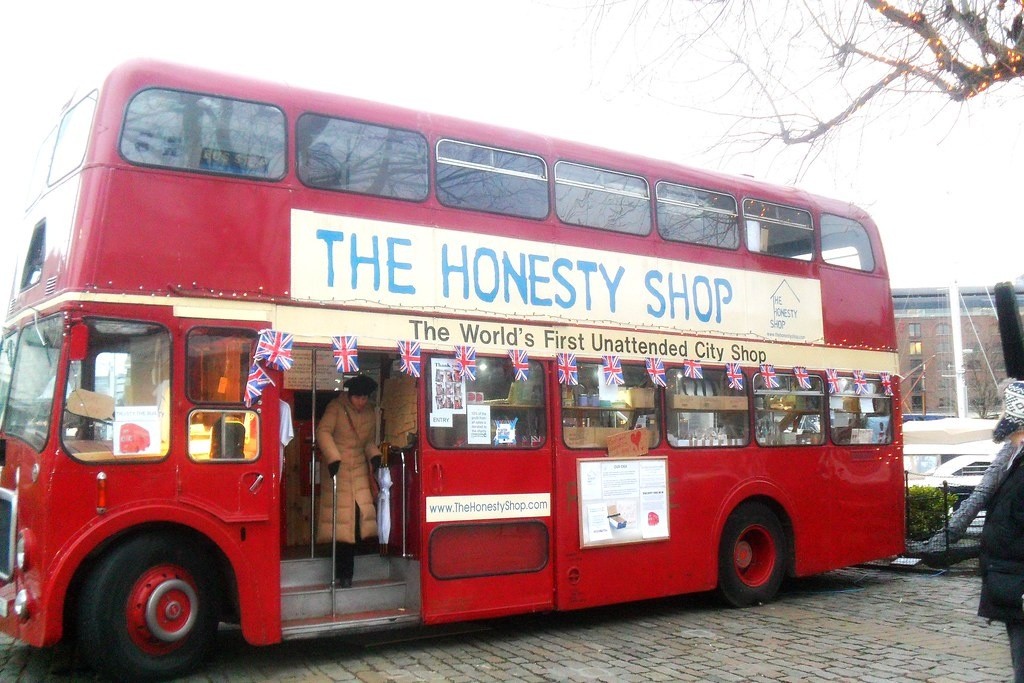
[315,374,383,589]
[975,382,1024,683]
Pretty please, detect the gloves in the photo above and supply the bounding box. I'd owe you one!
[328,461,339,478]
[370,456,381,472]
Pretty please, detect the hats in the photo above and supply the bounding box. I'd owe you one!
[344,376,378,394]
[992,378,1024,444]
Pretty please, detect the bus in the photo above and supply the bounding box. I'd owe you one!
[0,54,906,683]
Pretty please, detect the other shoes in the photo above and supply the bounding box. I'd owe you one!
[336,580,351,589]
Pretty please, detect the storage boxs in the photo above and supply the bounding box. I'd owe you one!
[672,394,748,411]
[623,388,655,409]
[564,426,624,448]
